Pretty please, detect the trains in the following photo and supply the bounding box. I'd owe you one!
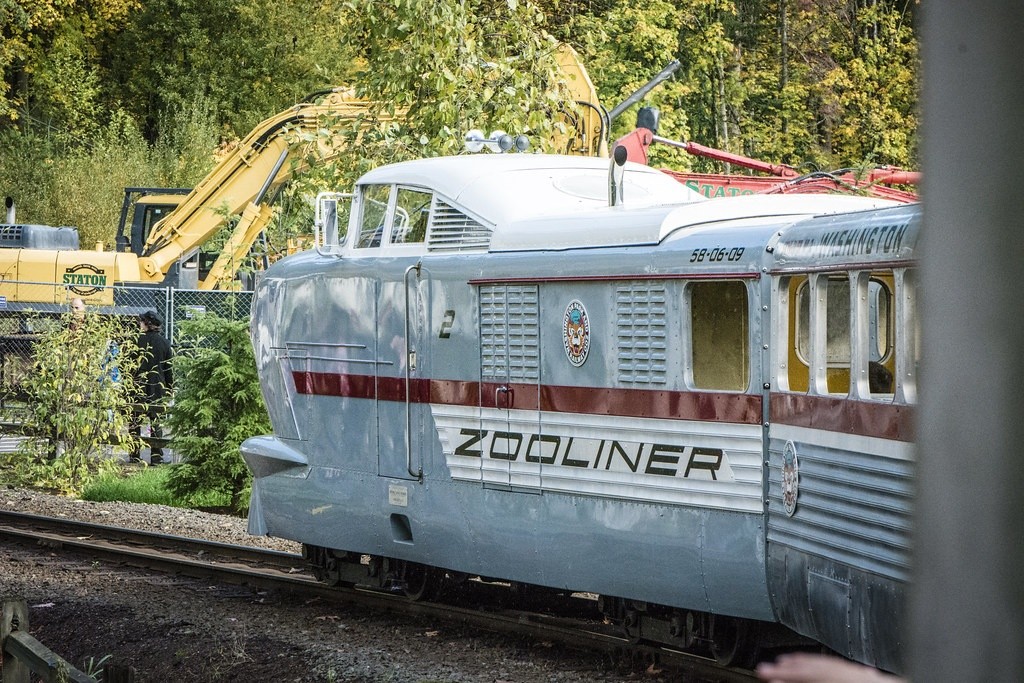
[239,57,922,677]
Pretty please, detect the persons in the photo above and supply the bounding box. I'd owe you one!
[124,310,173,466]
[43,299,124,457]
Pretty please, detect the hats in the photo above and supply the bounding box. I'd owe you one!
[140,311,163,326]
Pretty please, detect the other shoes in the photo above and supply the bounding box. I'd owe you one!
[152,460,160,465]
[132,457,140,462]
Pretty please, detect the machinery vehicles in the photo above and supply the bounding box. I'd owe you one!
[1,20,611,372]
